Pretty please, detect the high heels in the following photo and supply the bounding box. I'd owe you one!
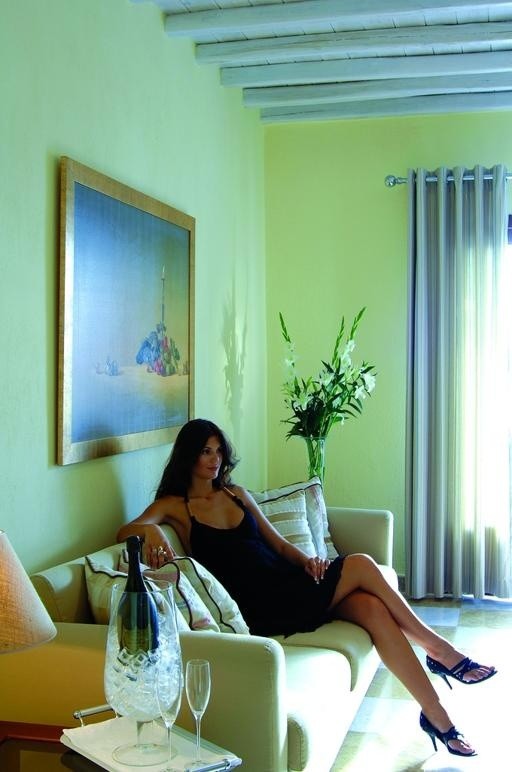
[419,708,479,759]
[424,643,497,689]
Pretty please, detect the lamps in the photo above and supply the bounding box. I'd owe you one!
[0,529,57,654]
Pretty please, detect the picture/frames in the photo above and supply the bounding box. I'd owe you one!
[57,156,196,466]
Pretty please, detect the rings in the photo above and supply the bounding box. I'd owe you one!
[152,549,158,556]
[157,546,166,556]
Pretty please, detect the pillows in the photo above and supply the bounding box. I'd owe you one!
[85,548,250,634]
[246,475,340,560]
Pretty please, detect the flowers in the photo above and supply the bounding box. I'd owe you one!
[278,306,379,476]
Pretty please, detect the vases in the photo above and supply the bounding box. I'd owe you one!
[305,437,327,494]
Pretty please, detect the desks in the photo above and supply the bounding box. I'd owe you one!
[0,721,107,772]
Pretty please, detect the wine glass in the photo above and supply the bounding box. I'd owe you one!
[102,581,181,768]
[158,659,184,768]
[184,657,214,770]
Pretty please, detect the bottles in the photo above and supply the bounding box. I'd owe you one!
[117,536,159,682]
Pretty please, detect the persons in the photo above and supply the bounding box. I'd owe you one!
[115,418,499,758]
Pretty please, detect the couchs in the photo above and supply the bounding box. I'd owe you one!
[0,507,399,772]
